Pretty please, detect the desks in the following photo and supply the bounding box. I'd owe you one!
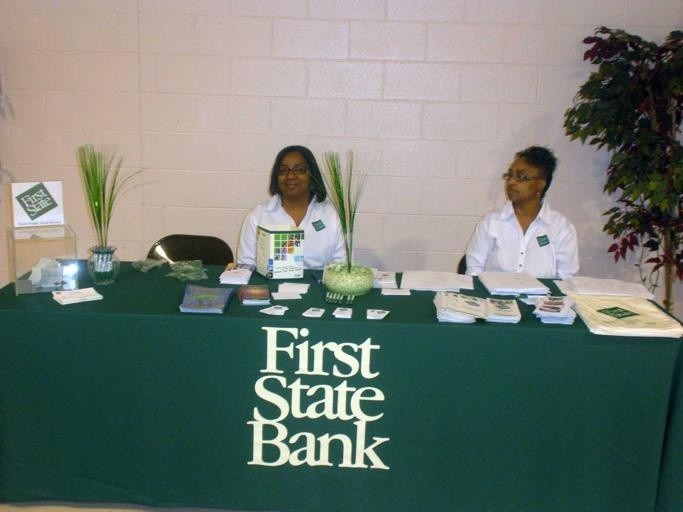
[1,259,683,512]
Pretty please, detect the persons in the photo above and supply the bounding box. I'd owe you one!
[237,145,348,271]
[465,145,580,279]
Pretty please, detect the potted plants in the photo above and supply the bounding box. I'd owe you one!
[319,146,375,297]
[75,145,144,287]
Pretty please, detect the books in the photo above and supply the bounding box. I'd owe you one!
[433,289,522,323]
[51,286,103,305]
[179,283,232,314]
[240,285,270,305]
[256,225,302,279]
[573,294,683,339]
[479,271,554,295]
[373,270,397,288]
[218,263,254,285]
[533,292,576,325]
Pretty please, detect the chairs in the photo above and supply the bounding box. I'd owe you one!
[147,233,234,266]
[456,256,466,277]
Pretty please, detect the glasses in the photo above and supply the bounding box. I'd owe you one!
[275,164,316,176]
[501,172,550,182]
